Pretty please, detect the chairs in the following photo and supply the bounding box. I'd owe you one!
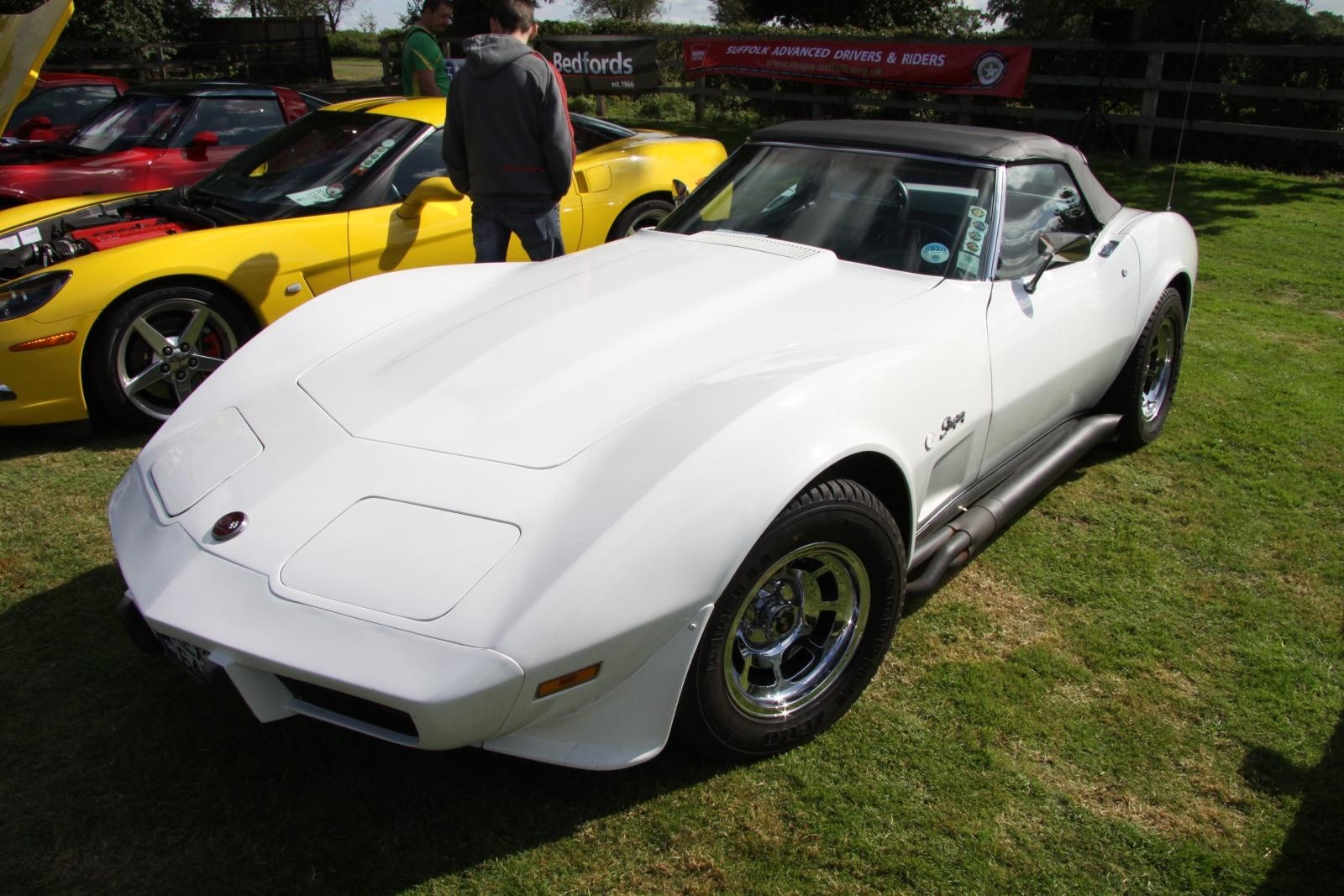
[810,173,910,263]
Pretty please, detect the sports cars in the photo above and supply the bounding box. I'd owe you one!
[0,90,736,436]
[103,120,1199,775]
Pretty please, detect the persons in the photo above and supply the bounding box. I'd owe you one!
[401,0,453,97]
[441,0,577,264]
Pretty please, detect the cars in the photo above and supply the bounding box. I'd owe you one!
[0,0,365,208]
